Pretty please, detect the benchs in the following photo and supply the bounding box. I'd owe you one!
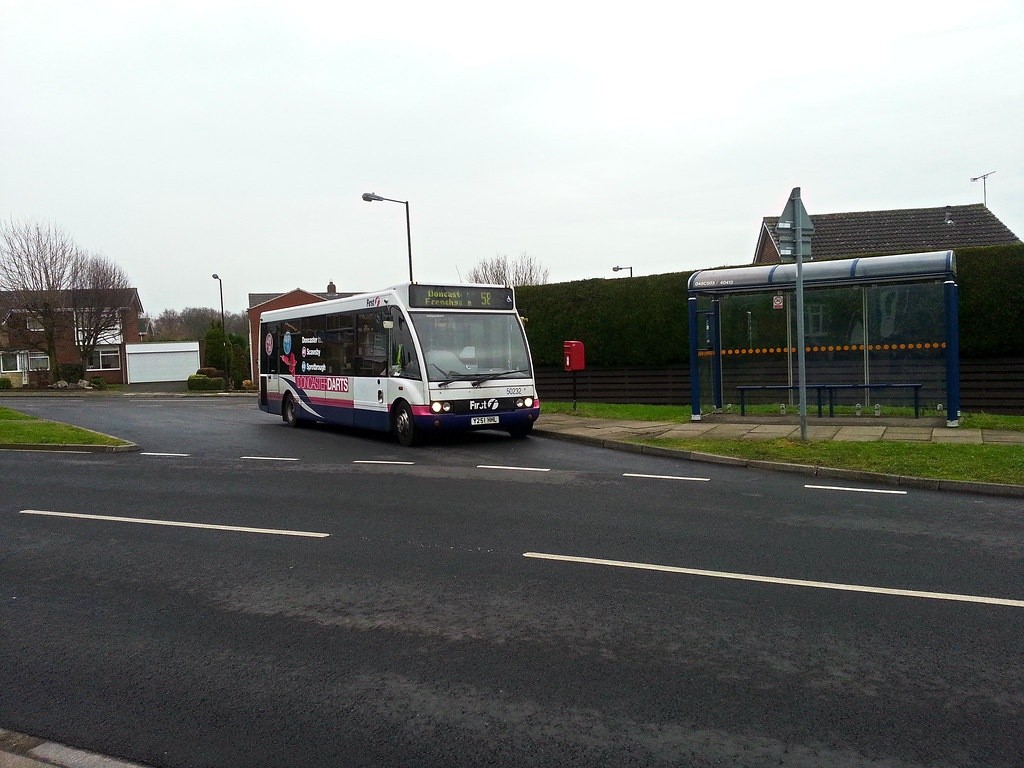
[826,383,922,420]
[736,384,824,418]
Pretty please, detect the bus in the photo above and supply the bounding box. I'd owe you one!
[257,281,541,447]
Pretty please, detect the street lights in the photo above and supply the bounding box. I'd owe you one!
[613,266,632,277]
[363,192,413,282]
[212,274,229,392]
